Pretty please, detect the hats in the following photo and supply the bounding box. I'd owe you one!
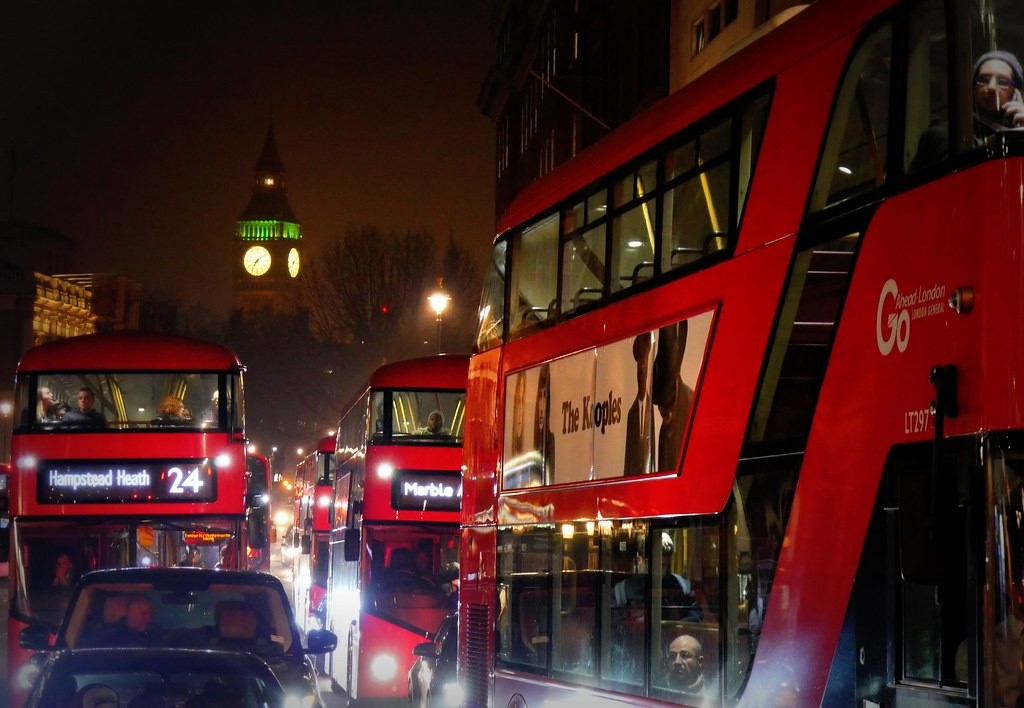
[630,332,652,361]
[428,410,444,429]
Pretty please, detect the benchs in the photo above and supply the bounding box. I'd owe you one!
[507,570,746,706]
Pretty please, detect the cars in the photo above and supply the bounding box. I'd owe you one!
[24,645,294,708]
[17,565,339,707]
[409,611,458,708]
[281,525,294,565]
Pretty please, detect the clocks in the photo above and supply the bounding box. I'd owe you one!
[244,246,271,276]
[288,248,300,277]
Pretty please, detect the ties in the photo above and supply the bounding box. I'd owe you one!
[640,401,645,435]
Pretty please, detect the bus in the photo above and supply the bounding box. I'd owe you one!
[321,353,646,708]
[456,0,1024,708]
[128,453,277,579]
[292,436,336,639]
[1,327,269,708]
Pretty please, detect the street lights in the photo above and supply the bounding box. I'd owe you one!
[425,272,451,352]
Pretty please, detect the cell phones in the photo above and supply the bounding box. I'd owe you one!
[1003,94,1017,127]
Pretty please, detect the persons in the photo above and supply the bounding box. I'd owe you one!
[36,386,107,428]
[905,51,1024,175]
[624,319,694,475]
[178,546,206,567]
[505,363,555,490]
[663,635,710,696]
[151,396,193,427]
[415,550,428,570]
[50,553,77,587]
[613,532,704,645]
[411,410,451,442]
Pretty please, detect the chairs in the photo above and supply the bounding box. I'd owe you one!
[104,595,127,623]
[521,230,722,333]
[214,601,257,642]
